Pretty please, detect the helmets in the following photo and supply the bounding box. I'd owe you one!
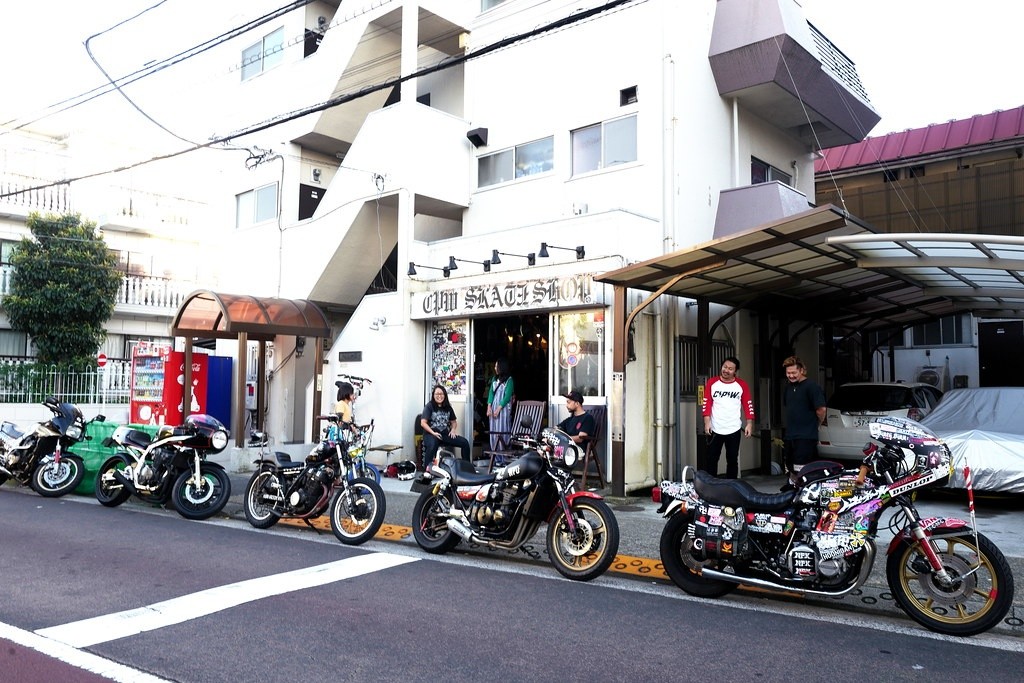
[397,461,416,480]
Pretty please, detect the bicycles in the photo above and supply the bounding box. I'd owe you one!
[323,417,381,485]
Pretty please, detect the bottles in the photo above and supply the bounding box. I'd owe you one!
[134,357,164,399]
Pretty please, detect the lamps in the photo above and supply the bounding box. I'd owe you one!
[407,242,586,277]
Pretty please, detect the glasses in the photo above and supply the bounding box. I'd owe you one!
[434,393,444,396]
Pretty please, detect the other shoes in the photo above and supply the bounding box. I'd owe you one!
[333,482,342,488]
[417,472,433,480]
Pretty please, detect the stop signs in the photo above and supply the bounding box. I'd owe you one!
[98,354,106,366]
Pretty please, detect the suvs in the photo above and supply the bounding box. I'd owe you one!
[816,380,944,462]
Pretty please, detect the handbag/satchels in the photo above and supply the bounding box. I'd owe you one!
[798,461,844,486]
[384,463,397,477]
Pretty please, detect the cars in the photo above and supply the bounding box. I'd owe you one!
[917,386,1024,508]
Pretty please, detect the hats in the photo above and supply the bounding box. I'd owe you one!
[562,391,584,404]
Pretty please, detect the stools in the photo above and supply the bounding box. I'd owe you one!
[369,444,402,478]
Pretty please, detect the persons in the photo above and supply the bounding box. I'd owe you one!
[421,385,480,474]
[487,360,514,467]
[779,355,826,491]
[554,391,594,461]
[702,357,755,479]
[333,382,361,489]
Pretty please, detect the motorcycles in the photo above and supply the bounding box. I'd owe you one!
[245,414,387,546]
[655,413,1014,636]
[0,394,108,498]
[95,413,232,520]
[412,414,620,582]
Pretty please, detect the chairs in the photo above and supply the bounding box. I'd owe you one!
[572,405,607,490]
[414,414,454,472]
[485,400,550,473]
